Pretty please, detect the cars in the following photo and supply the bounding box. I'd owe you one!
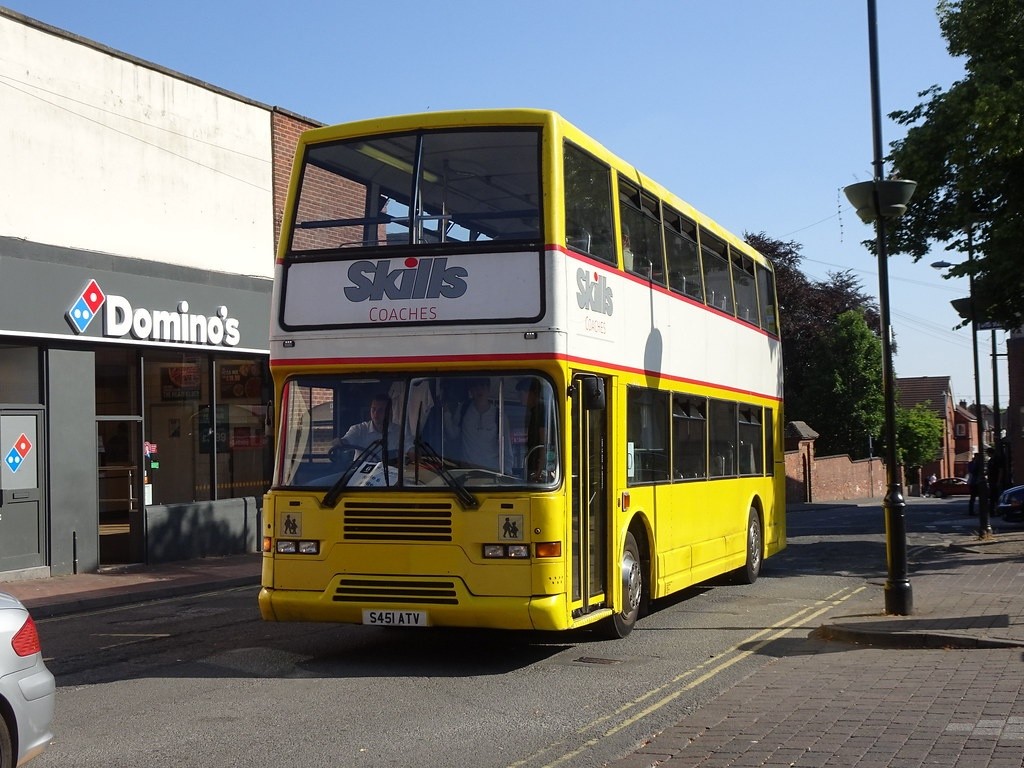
[0,587,59,768]
[996,485,1024,523]
[928,477,973,498]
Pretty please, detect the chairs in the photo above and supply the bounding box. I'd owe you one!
[634,440,733,479]
[493,230,749,321]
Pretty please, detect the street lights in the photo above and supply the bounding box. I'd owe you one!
[842,1,919,616]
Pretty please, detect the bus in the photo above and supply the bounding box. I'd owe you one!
[259,106,788,642]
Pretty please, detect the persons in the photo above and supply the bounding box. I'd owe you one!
[444,377,513,475]
[967,453,988,516]
[984,448,1004,517]
[621,222,634,270]
[925,475,931,492]
[931,473,937,483]
[332,393,415,463]
[515,377,546,474]
